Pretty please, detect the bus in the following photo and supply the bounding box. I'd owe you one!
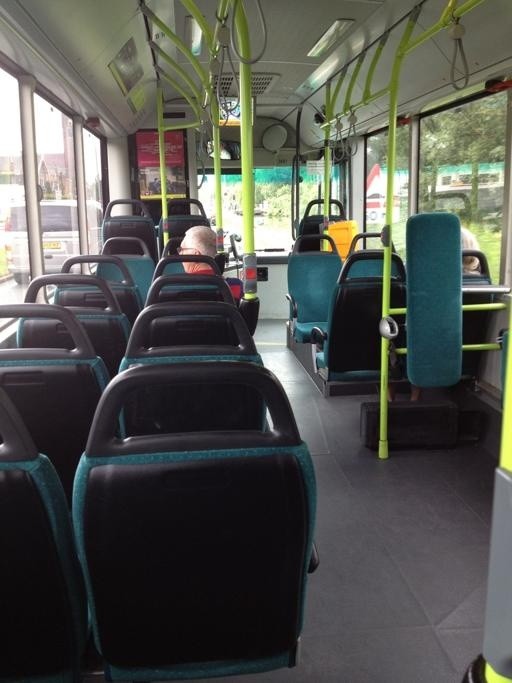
[354,161,505,227]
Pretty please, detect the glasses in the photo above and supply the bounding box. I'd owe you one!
[177,247,201,255]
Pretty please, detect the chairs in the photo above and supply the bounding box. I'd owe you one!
[285,198,493,396]
[1,197,320,681]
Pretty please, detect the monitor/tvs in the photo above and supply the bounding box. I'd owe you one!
[219,95,256,127]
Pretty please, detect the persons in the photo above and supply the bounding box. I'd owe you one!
[178,224,222,274]
[461,229,482,273]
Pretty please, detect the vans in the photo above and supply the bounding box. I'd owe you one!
[4,197,107,286]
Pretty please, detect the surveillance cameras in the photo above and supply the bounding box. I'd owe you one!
[313,113,323,124]
